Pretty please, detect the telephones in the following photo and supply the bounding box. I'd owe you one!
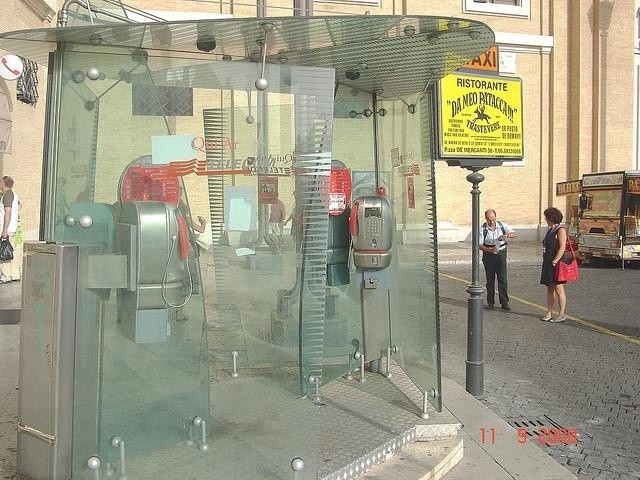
[176,210,203,296]
[348,196,392,270]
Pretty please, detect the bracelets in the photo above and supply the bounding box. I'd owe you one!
[506,234,509,238]
[552,259,557,265]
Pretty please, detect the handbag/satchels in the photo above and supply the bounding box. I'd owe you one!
[0,235,15,260]
[554,259,581,282]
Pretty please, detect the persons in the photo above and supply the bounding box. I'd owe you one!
[283,189,298,253]
[478,209,518,310]
[268,191,287,251]
[170,259,189,322]
[0,176,20,284]
[539,206,568,323]
[177,185,207,295]
[56,176,70,218]
[0,176,24,280]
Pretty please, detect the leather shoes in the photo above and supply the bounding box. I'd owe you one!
[541,315,552,321]
[550,316,566,323]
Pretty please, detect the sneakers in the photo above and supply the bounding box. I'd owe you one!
[501,303,512,310]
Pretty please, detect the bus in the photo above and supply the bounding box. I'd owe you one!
[577,169,640,265]
